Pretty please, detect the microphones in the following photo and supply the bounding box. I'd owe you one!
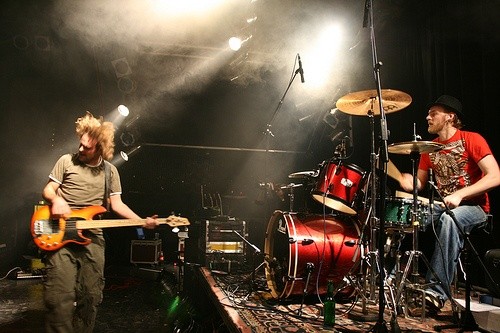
[427,167,434,212]
[298,56,304,83]
[363,0,370,27]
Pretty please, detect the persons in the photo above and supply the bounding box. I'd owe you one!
[401,94,500,308]
[42,114,158,333]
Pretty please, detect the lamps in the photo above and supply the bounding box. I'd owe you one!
[228,31,252,51]
[244,16,262,27]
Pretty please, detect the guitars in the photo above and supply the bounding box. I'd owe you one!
[30,205,191,252]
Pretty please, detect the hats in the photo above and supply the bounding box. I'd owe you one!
[430,94,462,115]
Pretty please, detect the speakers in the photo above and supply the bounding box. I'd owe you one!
[484,250,500,298]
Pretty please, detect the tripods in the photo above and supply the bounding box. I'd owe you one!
[316,103,494,333]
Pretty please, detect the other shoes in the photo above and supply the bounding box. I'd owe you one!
[423,290,444,308]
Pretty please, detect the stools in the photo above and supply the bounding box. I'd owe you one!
[434,222,491,333]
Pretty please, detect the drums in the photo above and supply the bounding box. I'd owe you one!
[365,197,429,235]
[309,156,370,216]
[263,209,366,302]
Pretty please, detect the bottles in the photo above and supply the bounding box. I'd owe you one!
[324,279,336,326]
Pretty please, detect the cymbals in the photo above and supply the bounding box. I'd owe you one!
[287,170,315,179]
[336,88,412,116]
[387,140,445,155]
